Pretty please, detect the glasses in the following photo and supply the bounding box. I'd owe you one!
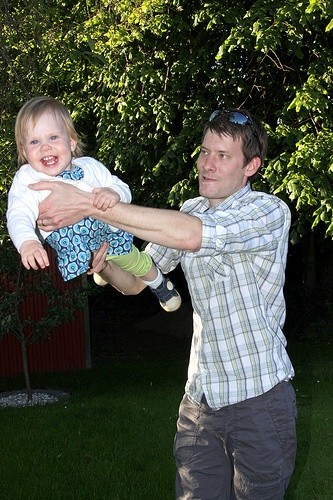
[208,107,265,153]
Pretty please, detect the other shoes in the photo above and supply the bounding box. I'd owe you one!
[153,272,182,314]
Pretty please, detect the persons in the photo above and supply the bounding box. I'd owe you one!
[5,96,182,314]
[27,109,300,499]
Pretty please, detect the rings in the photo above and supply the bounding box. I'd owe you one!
[42,219,45,227]
